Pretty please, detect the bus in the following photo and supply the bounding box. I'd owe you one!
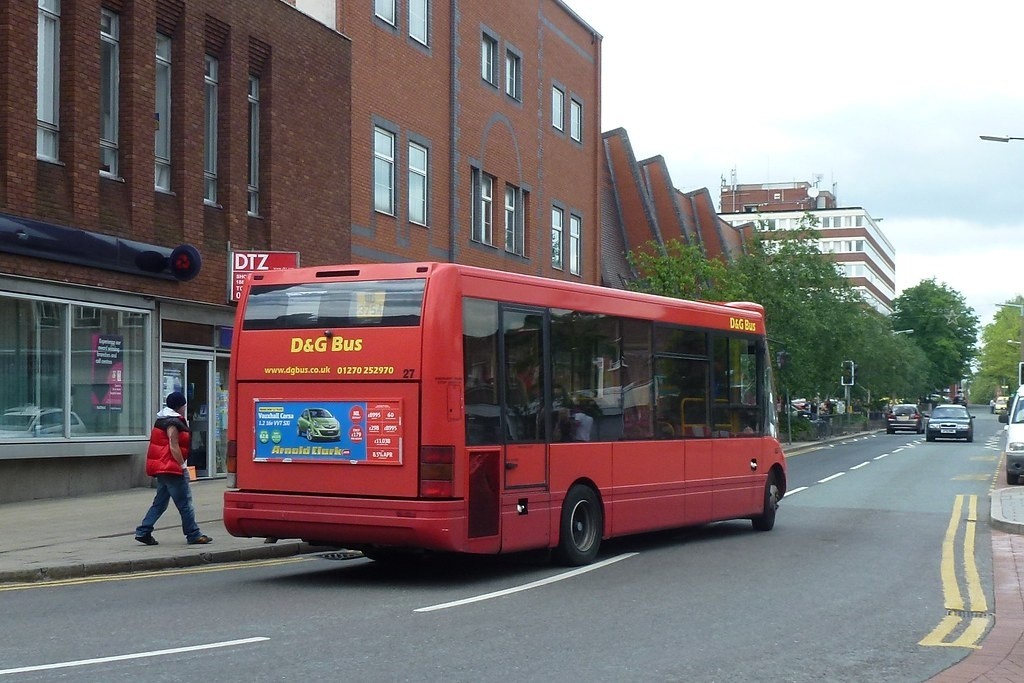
[222,261,787,567]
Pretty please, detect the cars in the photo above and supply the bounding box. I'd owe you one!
[777,398,844,420]
[927,394,951,403]
[953,393,967,408]
[925,404,976,442]
[886,404,925,434]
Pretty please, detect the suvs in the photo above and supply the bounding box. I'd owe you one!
[0,405,86,437]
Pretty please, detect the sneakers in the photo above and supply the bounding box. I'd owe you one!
[135,533,158,545]
[189,535,212,543]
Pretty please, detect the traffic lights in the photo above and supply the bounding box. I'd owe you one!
[840,360,855,385]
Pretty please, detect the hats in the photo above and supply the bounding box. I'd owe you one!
[167,392,186,408]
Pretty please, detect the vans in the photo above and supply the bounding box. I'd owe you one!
[998,384,1024,482]
[993,396,1009,414]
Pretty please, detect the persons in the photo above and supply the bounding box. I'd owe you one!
[552,385,597,442]
[821,400,834,415]
[990,399,996,414]
[853,399,863,412]
[810,398,817,413]
[134,392,213,545]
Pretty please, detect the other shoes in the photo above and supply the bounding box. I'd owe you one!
[265,537,277,543]
